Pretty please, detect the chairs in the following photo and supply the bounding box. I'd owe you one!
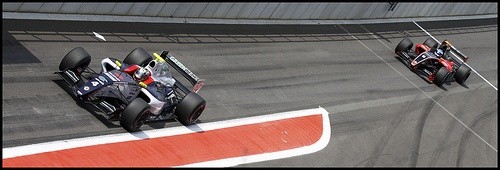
[143,75,154,85]
[440,46,448,59]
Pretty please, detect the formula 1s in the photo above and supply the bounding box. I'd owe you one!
[54,44,206,133]
[394,37,475,86]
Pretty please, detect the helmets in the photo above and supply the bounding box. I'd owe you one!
[133,67,151,82]
[434,48,444,58]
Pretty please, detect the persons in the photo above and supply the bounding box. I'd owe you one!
[133,66,151,82]
[434,48,443,57]
